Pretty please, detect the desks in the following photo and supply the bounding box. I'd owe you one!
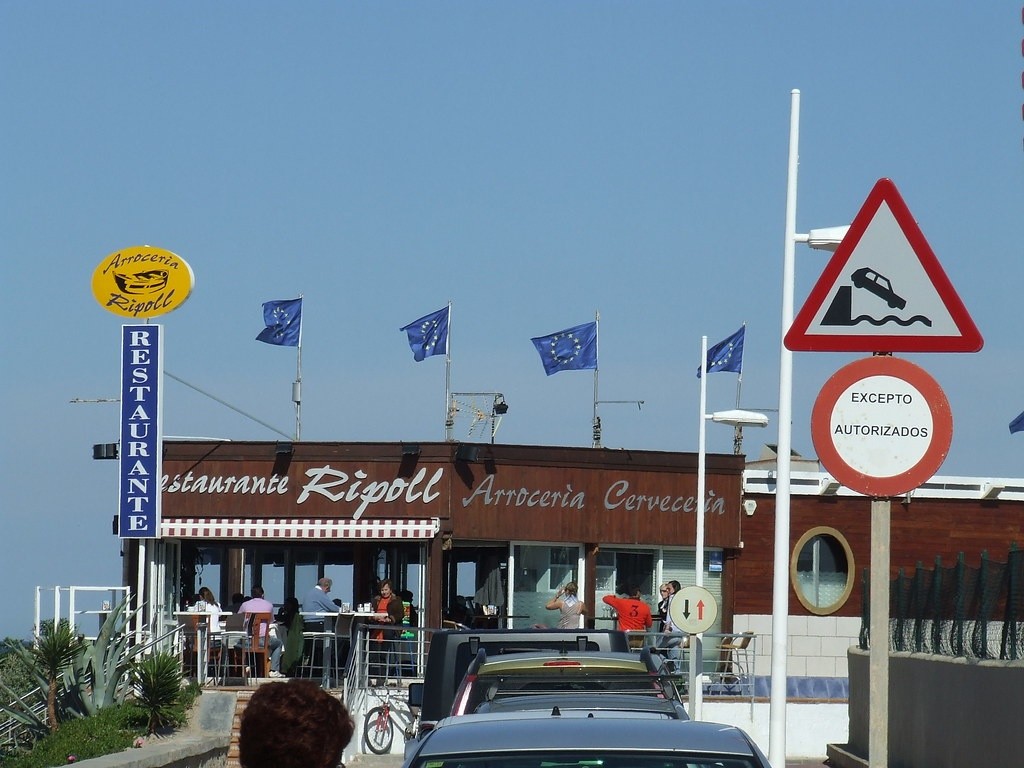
[592,617,619,628]
[299,611,388,689]
[474,615,530,630]
[173,612,233,684]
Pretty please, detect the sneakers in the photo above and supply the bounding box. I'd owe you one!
[269,670,285,678]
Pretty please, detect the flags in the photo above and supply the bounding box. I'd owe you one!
[255,293,304,347]
[1009,411,1024,434]
[530,309,599,376]
[697,319,746,379]
[400,299,452,362]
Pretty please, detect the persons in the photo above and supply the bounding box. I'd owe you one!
[183,587,222,649]
[445,598,483,625]
[546,582,589,629]
[239,679,356,768]
[603,583,652,632]
[221,584,299,678]
[656,580,684,674]
[369,578,419,676]
[302,578,342,632]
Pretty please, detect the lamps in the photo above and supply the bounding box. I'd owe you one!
[93,442,120,459]
[819,477,840,497]
[493,395,508,414]
[982,482,1005,501]
[454,440,480,463]
[401,442,422,456]
[276,440,292,454]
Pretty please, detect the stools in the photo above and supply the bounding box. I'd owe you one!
[178,601,414,688]
[708,631,753,695]
[444,620,459,629]
[627,629,690,690]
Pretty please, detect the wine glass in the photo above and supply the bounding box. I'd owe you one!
[602,604,610,618]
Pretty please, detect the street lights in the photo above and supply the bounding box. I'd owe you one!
[769,87,851,768]
[693,336,769,719]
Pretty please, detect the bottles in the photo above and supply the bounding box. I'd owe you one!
[357,603,364,613]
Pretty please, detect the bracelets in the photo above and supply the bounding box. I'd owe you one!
[555,594,559,598]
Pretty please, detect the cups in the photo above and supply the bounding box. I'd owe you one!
[188,607,195,612]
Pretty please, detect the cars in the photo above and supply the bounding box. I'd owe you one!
[404,636,773,768]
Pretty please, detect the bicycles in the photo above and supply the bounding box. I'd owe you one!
[364,688,421,754]
